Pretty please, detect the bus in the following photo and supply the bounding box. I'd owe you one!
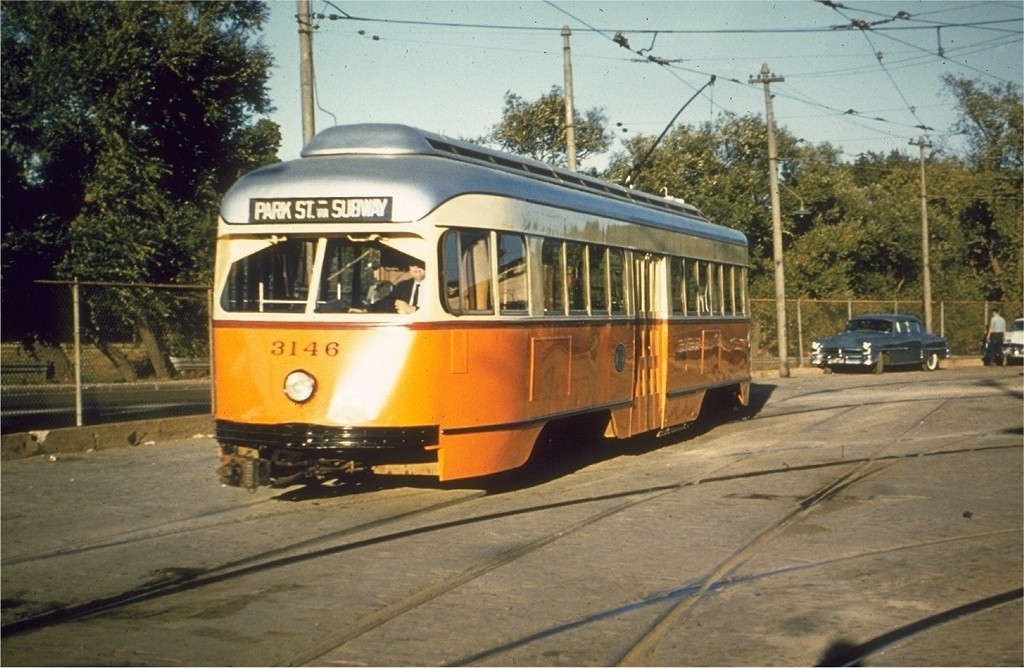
[208,121,754,490]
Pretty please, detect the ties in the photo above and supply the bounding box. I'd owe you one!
[413,284,420,305]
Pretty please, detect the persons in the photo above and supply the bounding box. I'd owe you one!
[982,309,1006,367]
[347,252,429,315]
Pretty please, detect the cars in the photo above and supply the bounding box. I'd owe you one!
[808,312,947,375]
[1000,318,1024,366]
